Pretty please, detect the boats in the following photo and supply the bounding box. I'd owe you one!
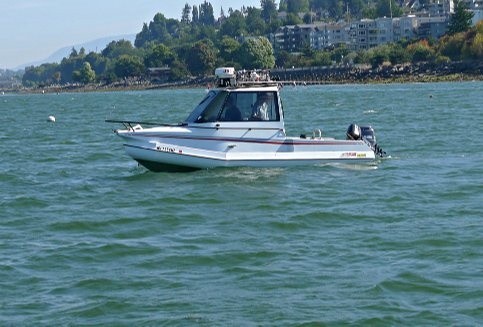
[102,67,389,174]
